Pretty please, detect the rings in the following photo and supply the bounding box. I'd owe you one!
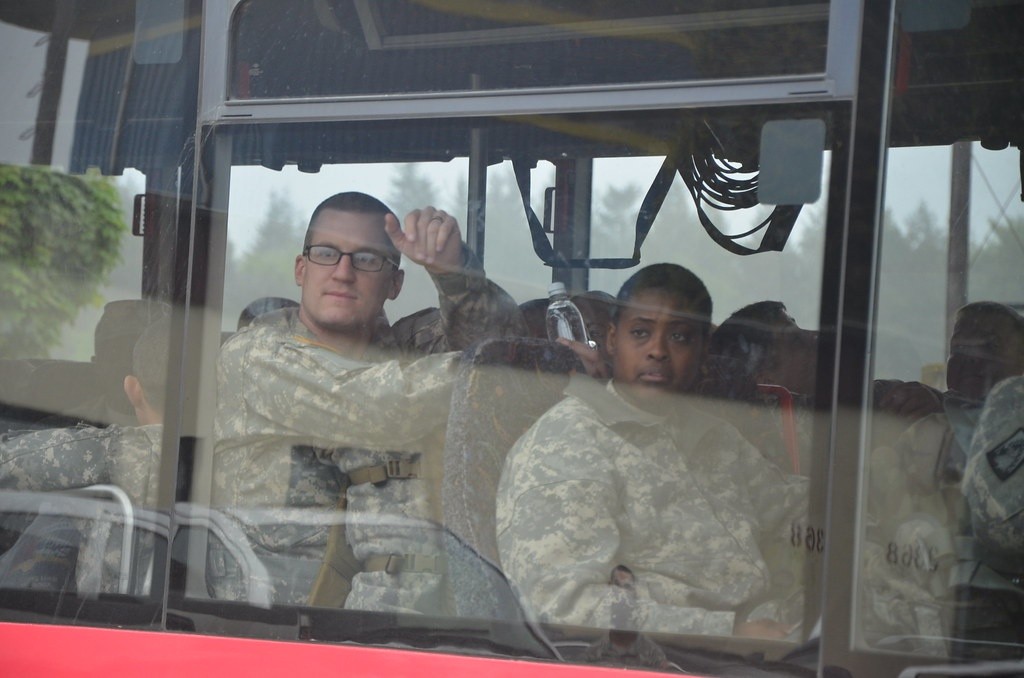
[432,215,444,225]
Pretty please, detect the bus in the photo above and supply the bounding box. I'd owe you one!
[1,0,1023,678]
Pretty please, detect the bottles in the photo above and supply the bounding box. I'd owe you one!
[546,281,597,351]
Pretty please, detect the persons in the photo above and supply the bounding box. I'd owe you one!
[494,262,1024,662]
[1,187,619,617]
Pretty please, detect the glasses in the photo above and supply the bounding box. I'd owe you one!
[304,245,399,272]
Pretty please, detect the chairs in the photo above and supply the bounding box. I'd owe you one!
[1,337,585,601]
